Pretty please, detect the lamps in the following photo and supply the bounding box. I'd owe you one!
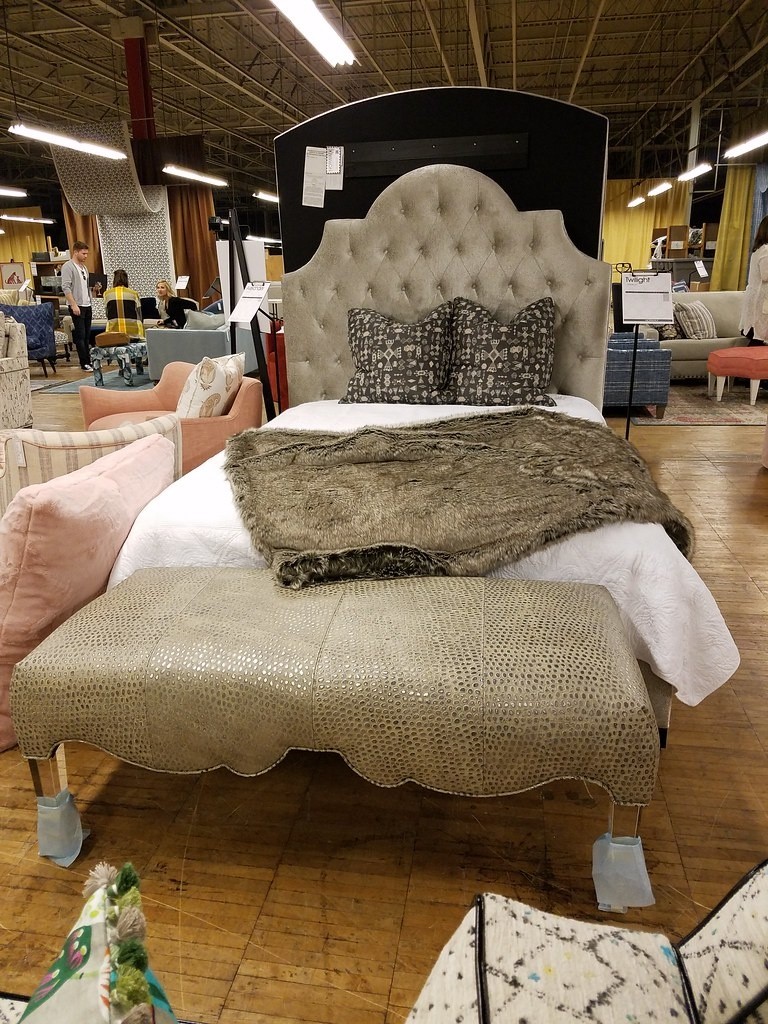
[0,0,363,224]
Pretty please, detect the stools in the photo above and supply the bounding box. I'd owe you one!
[706,346,768,406]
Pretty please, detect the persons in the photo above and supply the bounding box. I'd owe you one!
[61,242,99,372]
[156,280,197,329]
[51,263,61,294]
[738,214,768,390]
[103,269,144,377]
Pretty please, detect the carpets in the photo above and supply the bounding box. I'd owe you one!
[630,384,768,425]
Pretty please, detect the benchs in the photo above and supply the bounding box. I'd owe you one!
[63,297,199,350]
[145,324,266,386]
[11,569,661,912]
[90,342,147,386]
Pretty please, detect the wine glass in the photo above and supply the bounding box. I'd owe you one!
[96,282,102,295]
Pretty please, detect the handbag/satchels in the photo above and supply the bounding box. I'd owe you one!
[95,330,130,348]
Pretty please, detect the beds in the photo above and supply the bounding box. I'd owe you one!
[108,164,742,747]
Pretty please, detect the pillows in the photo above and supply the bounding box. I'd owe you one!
[177,352,246,418]
[1,434,181,1024]
[183,299,225,330]
[653,300,717,339]
[338,297,557,407]
[0,294,15,305]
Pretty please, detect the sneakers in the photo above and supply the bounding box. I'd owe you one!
[81,363,94,373]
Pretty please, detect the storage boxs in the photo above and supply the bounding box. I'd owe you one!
[689,282,710,292]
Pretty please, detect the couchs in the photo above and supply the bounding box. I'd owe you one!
[633,290,751,379]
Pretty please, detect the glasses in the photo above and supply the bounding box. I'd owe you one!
[81,271,87,280]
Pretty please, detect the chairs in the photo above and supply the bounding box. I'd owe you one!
[603,332,671,419]
[79,361,263,477]
[0,289,182,518]
